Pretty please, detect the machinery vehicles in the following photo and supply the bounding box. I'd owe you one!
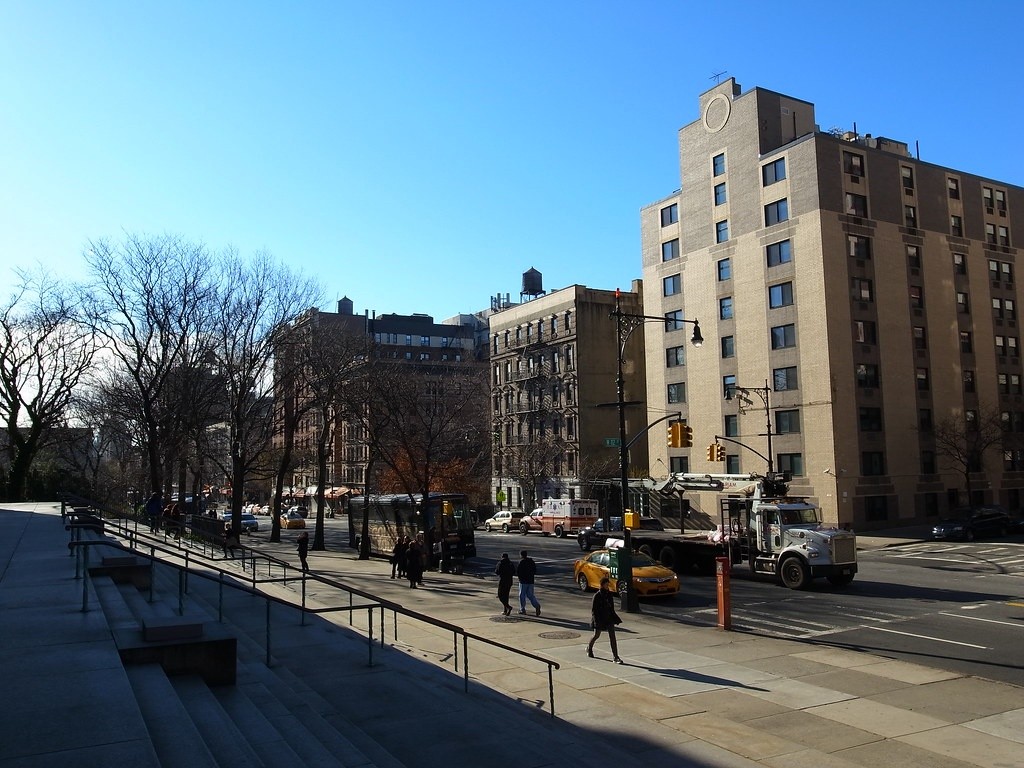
[594,469,860,593]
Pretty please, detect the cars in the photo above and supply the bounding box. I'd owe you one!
[932,507,1010,541]
[280,511,306,529]
[574,536,682,604]
[454,510,479,529]
[241,503,272,517]
[241,513,258,534]
[220,511,233,521]
[287,506,309,517]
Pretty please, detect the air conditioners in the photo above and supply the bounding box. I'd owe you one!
[552,434,559,440]
[554,401,557,406]
[569,435,573,440]
[566,330,570,335]
[494,471,499,475]
[567,400,572,404]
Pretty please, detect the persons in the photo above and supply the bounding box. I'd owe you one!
[390,533,425,588]
[516,550,541,616]
[296,531,309,571]
[585,577,624,665]
[221,523,235,558]
[143,491,161,534]
[493,553,516,615]
[162,504,190,535]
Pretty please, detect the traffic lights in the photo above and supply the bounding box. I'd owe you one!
[707,445,714,462]
[678,424,693,448]
[716,444,724,462]
[667,422,678,448]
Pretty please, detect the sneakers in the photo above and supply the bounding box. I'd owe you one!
[585,647,594,658]
[613,657,623,663]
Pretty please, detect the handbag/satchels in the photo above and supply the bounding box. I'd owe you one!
[613,613,622,625]
[390,557,394,564]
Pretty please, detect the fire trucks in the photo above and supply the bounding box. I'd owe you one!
[518,497,600,538]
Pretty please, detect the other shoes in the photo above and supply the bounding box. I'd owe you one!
[418,582,424,585]
[391,576,395,579]
[506,606,512,616]
[413,586,419,589]
[517,609,526,614]
[410,585,413,588]
[502,611,507,614]
[397,576,401,579]
[535,604,541,616]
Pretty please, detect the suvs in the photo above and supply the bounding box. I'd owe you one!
[578,516,665,552]
[485,510,526,533]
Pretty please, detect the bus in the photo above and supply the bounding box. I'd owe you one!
[348,490,477,570]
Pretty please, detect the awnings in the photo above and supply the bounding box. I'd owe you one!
[305,485,351,499]
[271,486,306,497]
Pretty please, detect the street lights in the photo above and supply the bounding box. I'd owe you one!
[607,287,706,614]
[724,378,773,476]
